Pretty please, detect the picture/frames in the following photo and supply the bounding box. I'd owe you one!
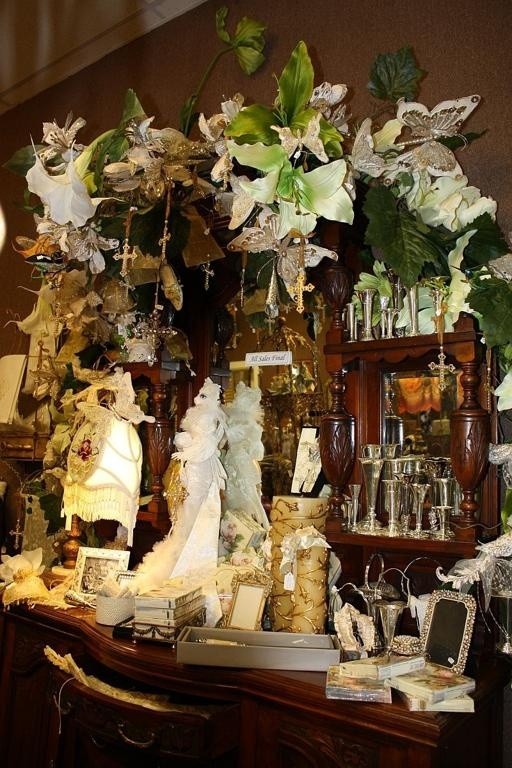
[421,590,476,679]
[73,546,132,600]
[113,571,137,590]
[223,573,273,630]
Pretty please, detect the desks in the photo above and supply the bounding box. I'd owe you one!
[1,589,512,768]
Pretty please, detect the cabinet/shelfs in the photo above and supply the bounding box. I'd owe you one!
[328,320,492,552]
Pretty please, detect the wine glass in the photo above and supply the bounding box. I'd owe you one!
[342,282,421,342]
[342,443,456,541]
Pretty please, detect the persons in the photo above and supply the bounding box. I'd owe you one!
[82,567,94,591]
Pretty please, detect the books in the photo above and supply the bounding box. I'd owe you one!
[325,655,476,713]
